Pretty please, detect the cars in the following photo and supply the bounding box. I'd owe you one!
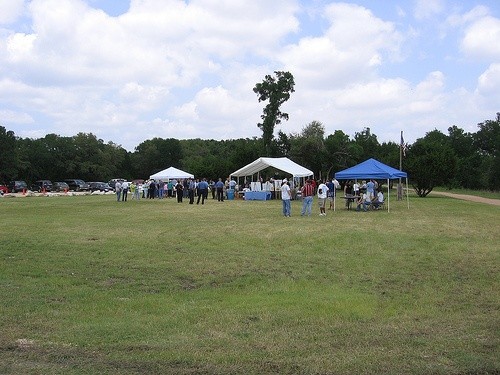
[53,182,69,192]
[0,184,9,193]
[88,182,114,192]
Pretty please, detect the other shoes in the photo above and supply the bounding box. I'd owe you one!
[320,213,323,216]
[323,213,326,216]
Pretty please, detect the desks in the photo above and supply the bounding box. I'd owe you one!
[244,191,272,200]
[339,196,361,210]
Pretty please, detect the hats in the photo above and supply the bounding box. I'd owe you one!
[282,179,288,183]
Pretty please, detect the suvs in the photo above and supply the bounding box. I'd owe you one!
[30,180,53,193]
[109,179,132,192]
[7,181,27,193]
[65,179,90,191]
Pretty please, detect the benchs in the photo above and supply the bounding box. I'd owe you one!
[366,201,386,210]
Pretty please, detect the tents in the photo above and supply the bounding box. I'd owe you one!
[229,157,314,189]
[150,166,194,179]
[334,158,409,213]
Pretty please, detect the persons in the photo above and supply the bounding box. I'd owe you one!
[260,176,263,183]
[275,172,280,180]
[327,179,336,210]
[266,175,270,181]
[217,178,224,202]
[332,178,339,197]
[225,178,229,189]
[130,178,177,200]
[311,179,316,196]
[301,179,315,216]
[318,180,329,216]
[231,178,236,189]
[281,178,292,218]
[210,180,216,199]
[176,178,208,205]
[115,180,129,201]
[344,179,385,211]
